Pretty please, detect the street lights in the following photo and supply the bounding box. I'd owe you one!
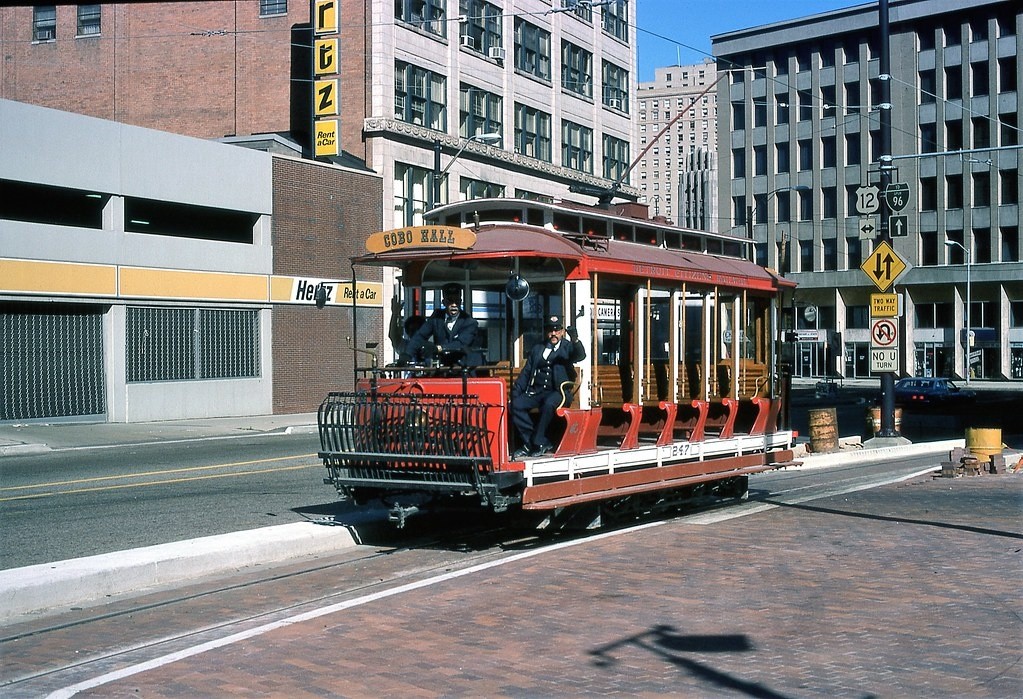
[742,185,808,262]
[945,240,972,384]
[432,133,502,311]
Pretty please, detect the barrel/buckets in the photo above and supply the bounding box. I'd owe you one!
[871,407,902,439]
[965,428,1002,462]
[808,406,840,453]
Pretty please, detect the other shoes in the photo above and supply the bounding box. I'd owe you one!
[514,443,535,458]
[532,440,553,457]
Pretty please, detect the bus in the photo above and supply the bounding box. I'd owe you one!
[315,70,801,537]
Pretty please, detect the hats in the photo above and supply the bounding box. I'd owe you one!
[544,316,563,328]
[441,283,462,302]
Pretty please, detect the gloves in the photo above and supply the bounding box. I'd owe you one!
[565,326,578,340]
[510,388,520,398]
[421,343,438,358]
[398,353,412,367]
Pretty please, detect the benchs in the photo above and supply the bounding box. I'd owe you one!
[490,359,584,448]
[705,363,736,440]
[589,366,633,443]
[641,364,674,446]
[735,360,771,435]
[671,364,708,442]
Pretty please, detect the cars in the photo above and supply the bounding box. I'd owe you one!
[891,378,977,413]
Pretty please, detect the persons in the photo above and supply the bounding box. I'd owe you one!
[396,282,482,378]
[508,314,586,458]
[388,294,435,368]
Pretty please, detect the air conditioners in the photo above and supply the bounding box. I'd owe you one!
[609,98,620,109]
[489,46,506,59]
[461,34,475,49]
[601,21,608,30]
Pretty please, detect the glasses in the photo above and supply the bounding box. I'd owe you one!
[546,325,562,332]
